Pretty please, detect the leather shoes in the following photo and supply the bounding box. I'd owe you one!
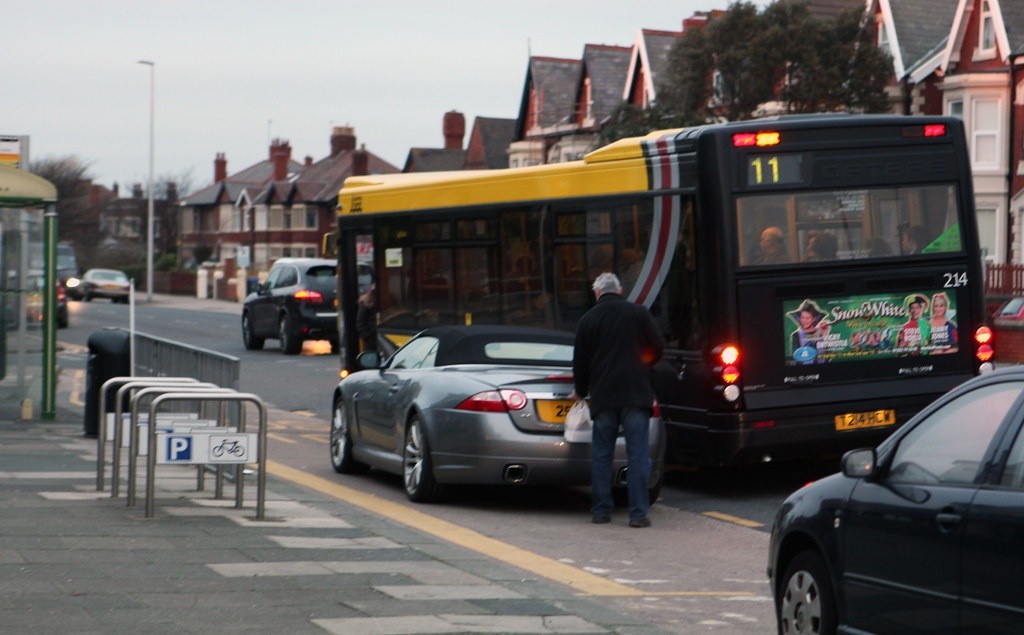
[592,508,611,523]
[629,516,651,528]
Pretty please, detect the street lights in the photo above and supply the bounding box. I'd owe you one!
[138,60,157,301]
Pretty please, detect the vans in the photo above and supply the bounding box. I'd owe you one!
[29,244,80,301]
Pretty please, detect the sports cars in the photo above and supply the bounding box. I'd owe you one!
[330,324,667,512]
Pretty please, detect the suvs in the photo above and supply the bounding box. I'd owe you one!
[242,258,375,355]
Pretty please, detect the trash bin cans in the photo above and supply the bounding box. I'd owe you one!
[84,326,130,440]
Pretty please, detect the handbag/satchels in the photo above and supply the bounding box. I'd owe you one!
[563,399,593,443]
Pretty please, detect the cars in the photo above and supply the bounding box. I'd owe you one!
[993,296,1024,321]
[767,365,1024,634]
[75,268,131,303]
[7,269,69,329]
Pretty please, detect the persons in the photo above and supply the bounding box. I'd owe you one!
[927,292,959,355]
[807,231,837,260]
[353,221,692,363]
[865,238,890,257]
[758,226,789,264]
[902,226,931,254]
[572,272,667,528]
[894,295,931,356]
[790,305,825,364]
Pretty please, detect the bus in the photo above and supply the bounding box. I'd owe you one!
[324,111,995,490]
